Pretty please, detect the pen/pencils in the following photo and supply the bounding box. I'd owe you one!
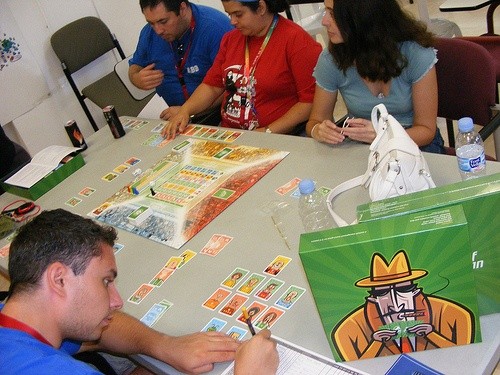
[242,308,256,336]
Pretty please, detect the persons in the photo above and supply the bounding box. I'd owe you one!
[0,208,280,375]
[127,0,235,127]
[305,0,444,155]
[161,0,323,137]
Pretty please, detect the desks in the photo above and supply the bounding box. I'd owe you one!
[1,116,500,375]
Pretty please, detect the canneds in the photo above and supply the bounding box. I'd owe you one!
[64,120,88,152]
[102,105,125,139]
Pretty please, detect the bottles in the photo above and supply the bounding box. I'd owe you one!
[298,178,336,232]
[455,117,486,181]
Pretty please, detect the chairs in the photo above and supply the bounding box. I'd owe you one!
[434,34,500,163]
[50,16,156,132]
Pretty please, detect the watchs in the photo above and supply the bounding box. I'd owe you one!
[265,125,272,134]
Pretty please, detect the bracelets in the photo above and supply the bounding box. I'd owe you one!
[311,123,320,138]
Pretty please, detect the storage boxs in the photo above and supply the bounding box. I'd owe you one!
[0,151,85,202]
[299,173,500,364]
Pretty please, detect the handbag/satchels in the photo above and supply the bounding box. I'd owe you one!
[326,103,436,230]
